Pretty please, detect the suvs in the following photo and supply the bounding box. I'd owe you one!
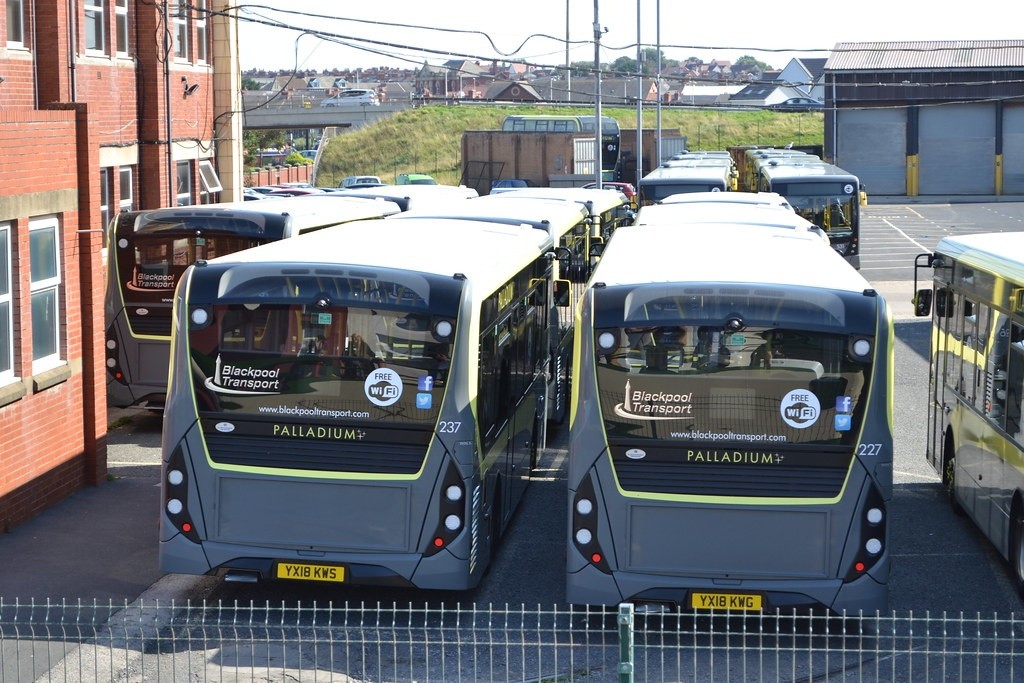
[396,172,438,185]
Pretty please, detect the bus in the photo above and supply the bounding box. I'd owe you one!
[638,149,740,207]
[102,179,477,411]
[500,115,622,170]
[156,183,629,594]
[741,146,866,270]
[912,231,1024,600]
[561,191,894,622]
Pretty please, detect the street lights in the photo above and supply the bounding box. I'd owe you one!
[550,78,556,100]
[472,78,475,89]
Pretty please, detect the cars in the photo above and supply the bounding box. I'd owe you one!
[495,180,540,188]
[319,88,381,107]
[582,183,635,201]
[299,150,318,160]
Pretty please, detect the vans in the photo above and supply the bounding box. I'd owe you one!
[336,176,383,188]
